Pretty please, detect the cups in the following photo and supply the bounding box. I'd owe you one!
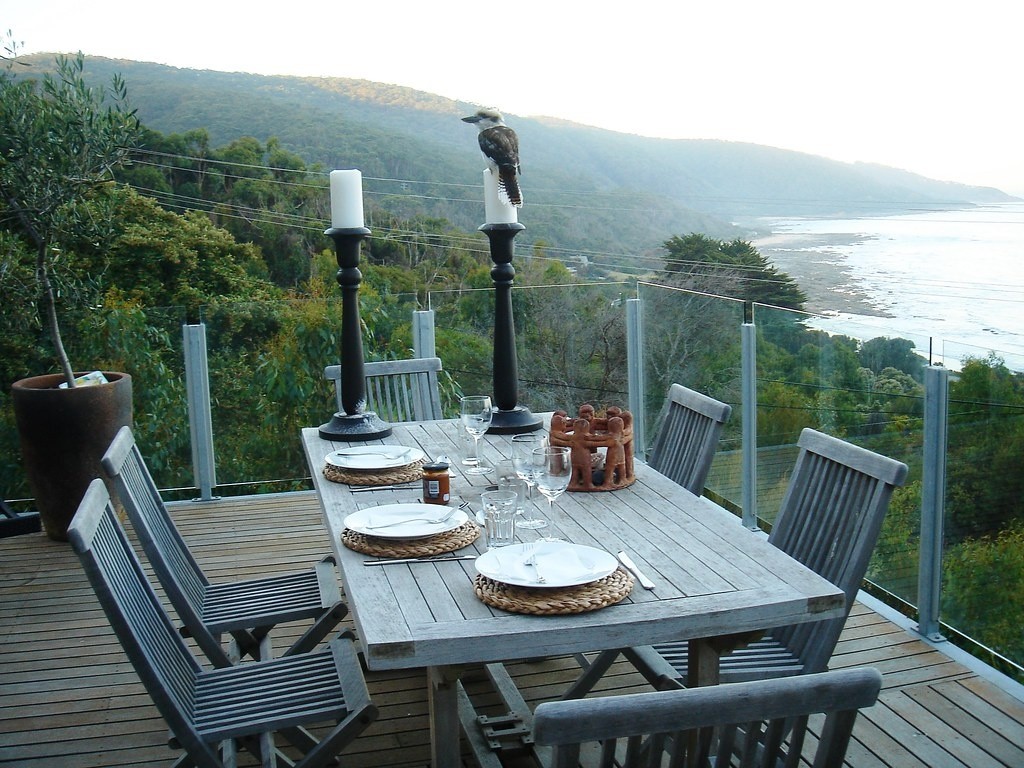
[495,460,526,515]
[482,490,517,547]
[457,417,483,465]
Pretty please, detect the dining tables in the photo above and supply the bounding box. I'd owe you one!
[300,409,846,768]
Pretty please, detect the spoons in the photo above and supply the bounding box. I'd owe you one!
[475,510,485,526]
[437,456,456,478]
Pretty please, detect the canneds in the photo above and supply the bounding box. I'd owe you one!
[421,462,450,505]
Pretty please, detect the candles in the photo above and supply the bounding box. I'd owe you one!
[329,168,364,229]
[483,167,518,224]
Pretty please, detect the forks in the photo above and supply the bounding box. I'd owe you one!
[336,449,411,460]
[522,543,545,582]
[366,506,458,529]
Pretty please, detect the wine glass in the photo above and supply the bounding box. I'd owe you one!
[533,447,572,543]
[511,434,550,529]
[461,395,494,475]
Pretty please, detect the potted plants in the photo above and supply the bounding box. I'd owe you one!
[0,30,150,543]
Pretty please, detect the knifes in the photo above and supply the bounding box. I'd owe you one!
[363,555,476,567]
[619,551,656,590]
[349,484,423,491]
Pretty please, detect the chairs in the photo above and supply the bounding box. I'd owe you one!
[100,426,349,670]
[523,383,732,702]
[620,427,908,768]
[324,357,443,423]
[65,477,380,768]
[531,664,884,768]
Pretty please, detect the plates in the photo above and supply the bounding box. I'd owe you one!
[344,504,468,540]
[325,445,424,470]
[474,541,618,587]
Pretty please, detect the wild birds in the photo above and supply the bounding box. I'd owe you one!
[462,106,524,208]
